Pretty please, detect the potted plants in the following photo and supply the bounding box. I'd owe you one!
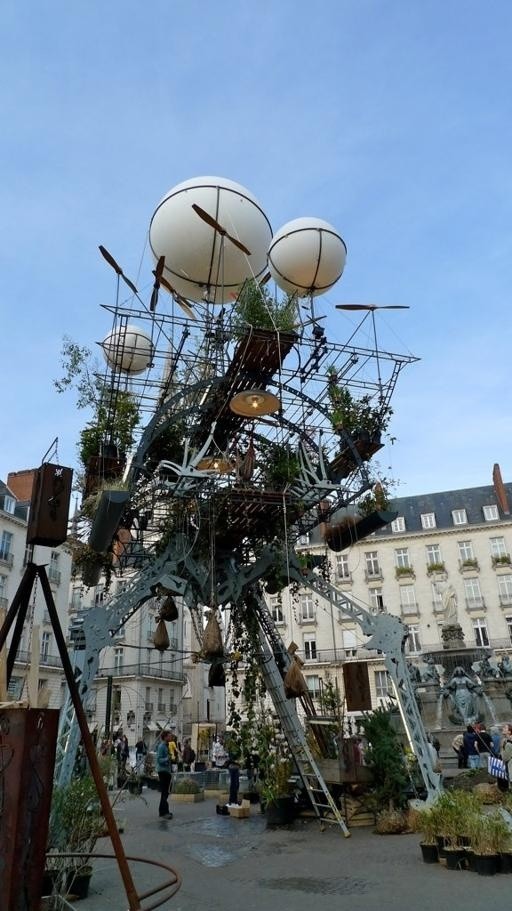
[323,367,384,458]
[74,336,134,588]
[413,788,511,875]
[262,744,297,825]
[45,763,141,901]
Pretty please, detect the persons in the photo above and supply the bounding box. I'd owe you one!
[156,728,174,821]
[452,724,511,794]
[102,735,229,775]
[225,730,242,805]
[442,665,488,720]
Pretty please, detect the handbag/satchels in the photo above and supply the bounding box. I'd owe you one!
[487,756,507,780]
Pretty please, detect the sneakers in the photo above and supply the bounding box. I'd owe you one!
[159,812,173,820]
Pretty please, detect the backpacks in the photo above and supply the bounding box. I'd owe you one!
[191,750,195,762]
